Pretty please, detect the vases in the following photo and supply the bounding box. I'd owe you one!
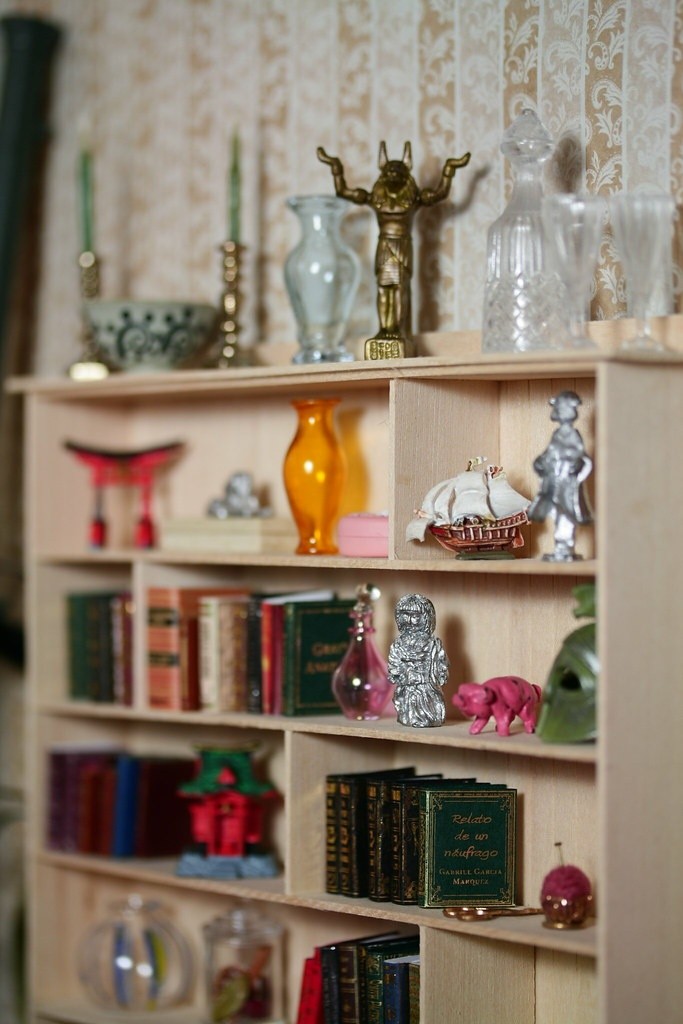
[282,195,363,365]
[283,397,349,557]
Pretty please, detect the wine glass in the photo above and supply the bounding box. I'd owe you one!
[541,196,602,349]
[608,195,673,353]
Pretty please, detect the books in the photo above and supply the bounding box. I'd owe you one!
[147,586,358,712]
[296,928,421,1024]
[47,748,201,860]
[324,764,525,908]
[65,589,133,705]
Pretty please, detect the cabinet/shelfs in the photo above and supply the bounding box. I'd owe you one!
[0,313,683,1024]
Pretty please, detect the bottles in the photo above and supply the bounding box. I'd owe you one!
[482,109,564,352]
[331,594,392,720]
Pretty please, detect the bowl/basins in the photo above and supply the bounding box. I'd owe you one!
[78,299,218,372]
